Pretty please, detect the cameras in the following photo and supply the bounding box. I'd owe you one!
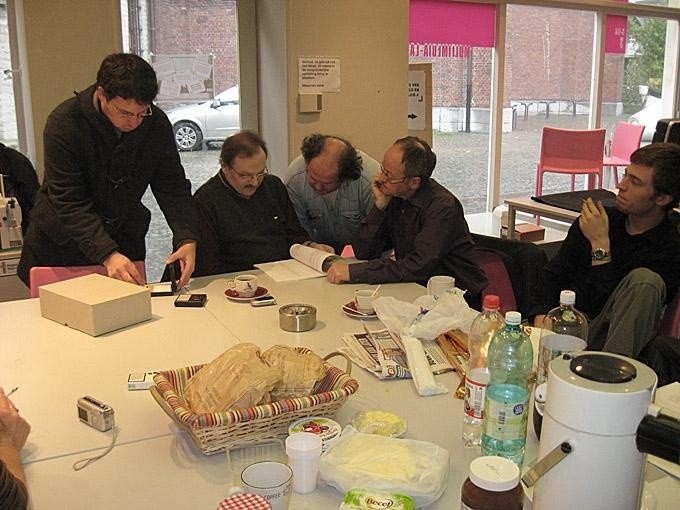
[77,394,116,432]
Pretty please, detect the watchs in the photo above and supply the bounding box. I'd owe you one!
[591,247,612,262]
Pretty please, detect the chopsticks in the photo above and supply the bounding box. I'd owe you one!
[436,328,470,381]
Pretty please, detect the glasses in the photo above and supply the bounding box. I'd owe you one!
[110,101,153,121]
[379,161,406,182]
[231,167,269,182]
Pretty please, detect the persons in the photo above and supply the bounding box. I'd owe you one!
[527,142,680,359]
[0,386,31,510]
[173,131,335,276]
[282,134,384,255]
[16,52,200,292]
[325,135,489,312]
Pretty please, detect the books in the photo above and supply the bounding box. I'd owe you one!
[252,243,336,282]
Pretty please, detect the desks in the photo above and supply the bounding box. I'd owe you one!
[504,189,680,241]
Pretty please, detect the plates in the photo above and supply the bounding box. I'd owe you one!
[224,286,268,301]
[342,301,378,319]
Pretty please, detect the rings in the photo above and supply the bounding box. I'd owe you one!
[9,405,20,414]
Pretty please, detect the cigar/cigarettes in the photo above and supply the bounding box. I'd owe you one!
[4,386,19,398]
[579,196,589,205]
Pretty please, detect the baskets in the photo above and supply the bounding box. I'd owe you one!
[149,347,358,456]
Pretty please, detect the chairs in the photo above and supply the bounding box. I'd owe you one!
[472,250,517,316]
[31,260,147,297]
[593,120,646,190]
[533,126,606,227]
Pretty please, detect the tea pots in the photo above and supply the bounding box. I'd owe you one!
[520,350,659,510]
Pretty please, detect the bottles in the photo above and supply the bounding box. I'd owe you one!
[535,290,588,388]
[480,311,533,468]
[461,295,504,448]
[460,455,525,510]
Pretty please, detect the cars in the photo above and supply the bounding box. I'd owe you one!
[165,84,240,151]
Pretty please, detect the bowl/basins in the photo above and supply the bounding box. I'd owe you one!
[288,416,342,452]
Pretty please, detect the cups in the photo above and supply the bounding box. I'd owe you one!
[353,290,380,315]
[428,276,455,297]
[285,433,323,495]
[227,460,293,510]
[227,274,258,298]
